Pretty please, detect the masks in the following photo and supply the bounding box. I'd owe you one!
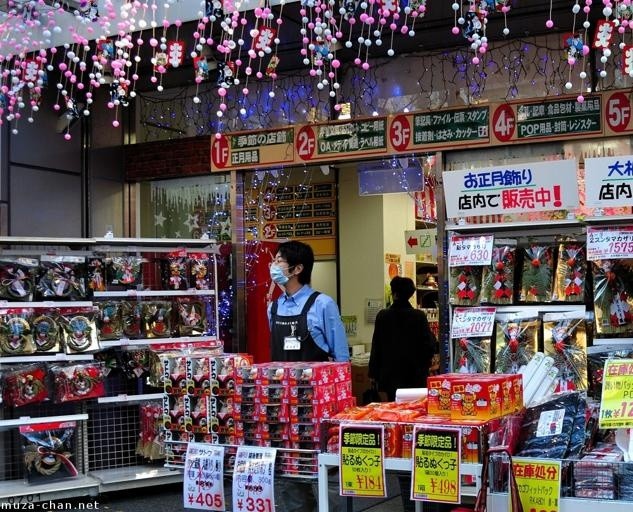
[268,265,294,285]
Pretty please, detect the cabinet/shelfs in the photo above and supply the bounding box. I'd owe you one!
[0,236,99,507]
[415,261,440,315]
[442,219,587,394]
[486,452,633,512]
[88,238,222,494]
[583,214,633,403]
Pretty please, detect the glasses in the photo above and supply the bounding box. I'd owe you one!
[270,257,288,263]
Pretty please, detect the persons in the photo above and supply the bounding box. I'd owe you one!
[368,275,439,402]
[268,241,350,511]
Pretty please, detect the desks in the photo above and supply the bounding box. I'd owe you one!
[317,452,485,512]
